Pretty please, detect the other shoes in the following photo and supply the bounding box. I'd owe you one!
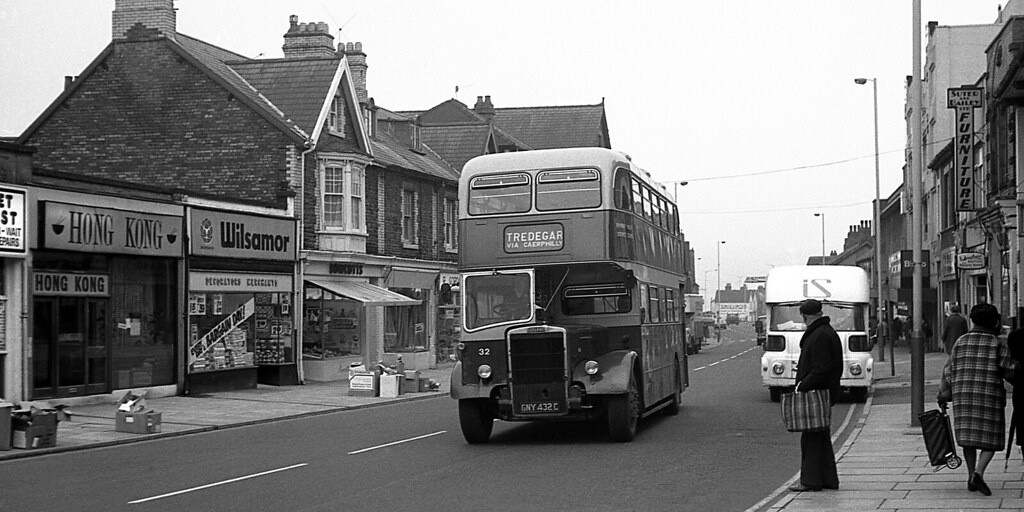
[971,471,992,496]
[968,479,977,491]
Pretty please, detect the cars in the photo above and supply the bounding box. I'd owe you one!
[726,312,739,326]
[754,316,766,345]
[739,313,747,322]
[703,314,715,323]
[714,319,727,329]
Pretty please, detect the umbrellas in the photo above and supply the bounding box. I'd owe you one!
[1004,411,1015,473]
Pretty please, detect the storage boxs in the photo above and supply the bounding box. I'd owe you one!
[380,370,430,398]
[115,408,162,435]
[12,404,72,451]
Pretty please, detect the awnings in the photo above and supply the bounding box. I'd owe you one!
[306,278,422,307]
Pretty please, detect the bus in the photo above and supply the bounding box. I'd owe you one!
[441,148,691,442]
[755,266,881,401]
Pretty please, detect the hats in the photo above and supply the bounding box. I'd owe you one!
[969,303,999,326]
[799,299,822,315]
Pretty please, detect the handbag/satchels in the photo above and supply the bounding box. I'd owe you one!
[779,380,832,433]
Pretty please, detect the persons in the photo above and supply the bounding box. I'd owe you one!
[1007,326,1024,462]
[497,275,533,320]
[788,299,843,492]
[871,302,968,355]
[936,304,1011,496]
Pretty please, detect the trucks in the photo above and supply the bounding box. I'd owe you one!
[683,295,705,354]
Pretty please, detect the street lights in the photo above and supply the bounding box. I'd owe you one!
[718,241,725,324]
[674,181,688,204]
[814,213,824,264]
[704,269,718,310]
[856,78,884,362]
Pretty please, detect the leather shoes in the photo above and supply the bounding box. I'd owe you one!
[788,483,822,492]
[821,484,839,490]
